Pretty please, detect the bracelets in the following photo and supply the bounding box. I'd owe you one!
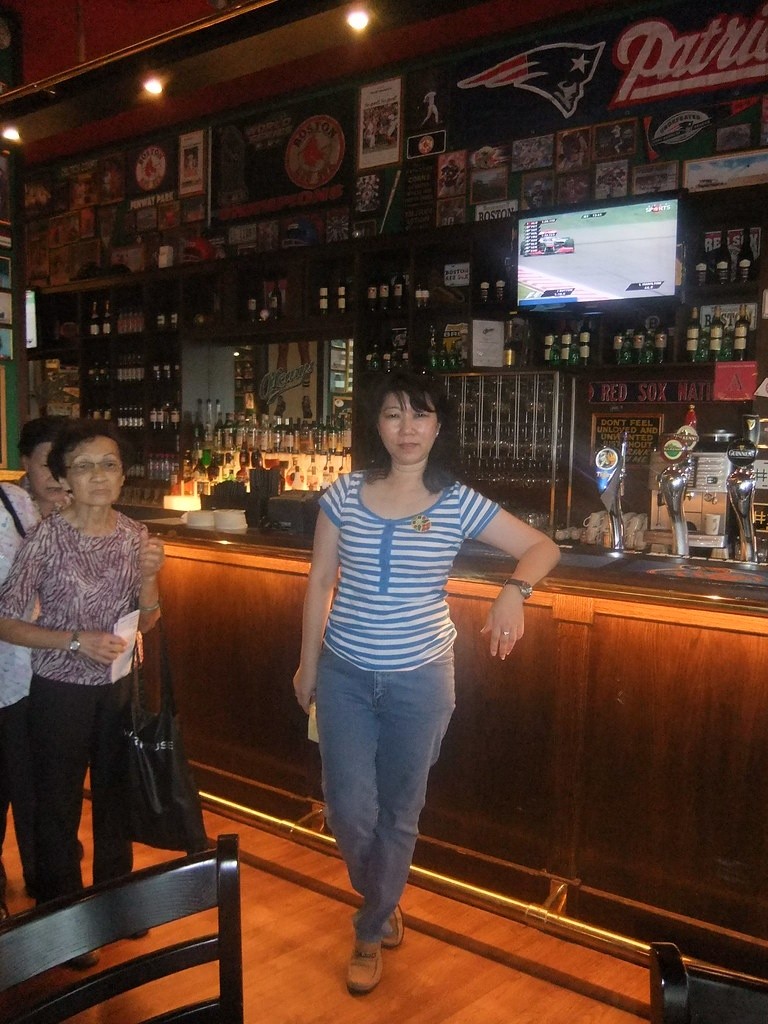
[138,601,160,611]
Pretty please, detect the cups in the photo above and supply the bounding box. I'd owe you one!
[556,510,649,550]
[185,508,247,530]
[704,513,721,535]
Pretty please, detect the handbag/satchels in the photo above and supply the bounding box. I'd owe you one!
[122,598,207,851]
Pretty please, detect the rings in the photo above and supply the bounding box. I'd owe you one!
[501,631,510,636]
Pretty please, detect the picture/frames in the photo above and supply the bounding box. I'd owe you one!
[355,75,406,173]
[176,128,207,200]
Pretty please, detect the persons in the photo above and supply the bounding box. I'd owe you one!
[0,416,168,969]
[293,365,561,997]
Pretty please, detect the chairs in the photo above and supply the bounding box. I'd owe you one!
[648,941,768,1024]
[0,833,245,1024]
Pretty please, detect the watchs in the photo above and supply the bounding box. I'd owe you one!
[69,631,80,658]
[503,577,533,599]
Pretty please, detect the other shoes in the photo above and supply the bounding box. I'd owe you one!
[61,949,100,966]
[382,901,404,948]
[347,938,382,994]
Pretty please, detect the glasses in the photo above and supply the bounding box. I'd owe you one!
[62,462,123,473]
[375,366,443,388]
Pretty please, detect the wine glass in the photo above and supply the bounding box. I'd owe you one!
[436,375,566,540]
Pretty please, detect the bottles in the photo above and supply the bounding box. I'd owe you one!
[684,403,697,431]
[314,257,515,317]
[541,319,598,369]
[614,318,667,364]
[46,299,146,340]
[363,318,532,374]
[685,305,750,361]
[153,282,285,334]
[31,343,354,500]
[692,226,754,285]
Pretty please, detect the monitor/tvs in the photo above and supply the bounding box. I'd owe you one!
[508,188,688,315]
[25,285,44,361]
